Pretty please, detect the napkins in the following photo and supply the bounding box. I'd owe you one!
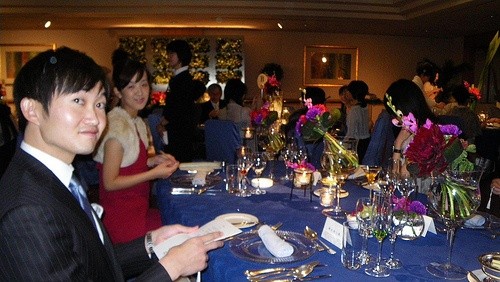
[190,170,207,186]
[469,214,486,227]
[257,224,294,260]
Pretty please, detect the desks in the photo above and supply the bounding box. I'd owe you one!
[151,159,500,282]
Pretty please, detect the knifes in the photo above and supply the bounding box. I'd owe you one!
[304,229,324,252]
[243,260,319,277]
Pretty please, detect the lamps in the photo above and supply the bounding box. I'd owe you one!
[276,16,287,30]
[40,16,51,28]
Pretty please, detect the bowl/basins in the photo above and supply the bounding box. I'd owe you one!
[478,252,500,280]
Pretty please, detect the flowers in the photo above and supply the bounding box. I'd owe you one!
[250,76,359,172]
[387,192,426,237]
[384,81,482,218]
[283,158,316,173]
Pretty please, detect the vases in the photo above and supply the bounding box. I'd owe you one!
[292,169,314,188]
[390,212,425,241]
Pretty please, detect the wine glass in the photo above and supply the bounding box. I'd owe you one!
[236,135,482,280]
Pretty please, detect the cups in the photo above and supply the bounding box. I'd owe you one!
[341,220,366,270]
[319,180,338,207]
[226,165,240,193]
[479,111,486,122]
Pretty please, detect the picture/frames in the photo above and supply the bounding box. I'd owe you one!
[0,43,58,86]
[303,44,359,87]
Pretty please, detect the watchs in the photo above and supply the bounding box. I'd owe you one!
[391,146,401,153]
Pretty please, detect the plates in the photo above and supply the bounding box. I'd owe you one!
[215,213,258,229]
[467,269,500,282]
[228,231,317,264]
[170,175,224,188]
[430,211,500,228]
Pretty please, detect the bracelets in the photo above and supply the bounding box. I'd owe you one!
[146,231,153,252]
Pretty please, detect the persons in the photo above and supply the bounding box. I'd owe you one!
[383,59,500,218]
[0,39,282,282]
[281,80,370,164]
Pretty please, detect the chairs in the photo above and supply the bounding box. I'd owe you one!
[146,107,394,167]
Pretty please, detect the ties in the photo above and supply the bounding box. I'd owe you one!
[69,173,97,231]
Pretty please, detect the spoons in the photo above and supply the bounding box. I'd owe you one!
[305,225,336,255]
[245,264,314,282]
